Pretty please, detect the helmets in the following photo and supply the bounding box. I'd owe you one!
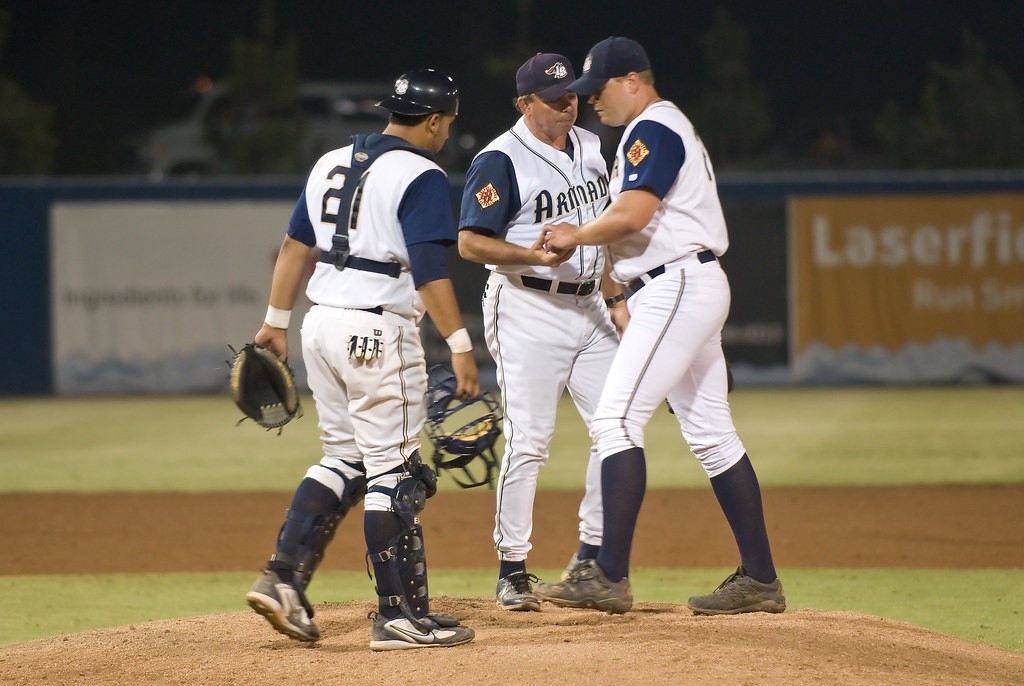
[373,68,459,116]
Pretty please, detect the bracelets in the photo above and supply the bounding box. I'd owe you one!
[605,292,626,306]
[445,327,474,354]
[263,304,292,330]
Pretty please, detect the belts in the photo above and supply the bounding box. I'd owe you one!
[624,250,716,300]
[313,300,384,316]
[522,274,602,296]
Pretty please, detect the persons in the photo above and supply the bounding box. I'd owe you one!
[458,51,630,612]
[530,34,787,614]
[245,68,481,651]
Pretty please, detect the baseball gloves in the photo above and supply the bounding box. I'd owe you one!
[225,338,307,434]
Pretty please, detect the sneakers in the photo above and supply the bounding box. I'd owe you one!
[368,612,475,651]
[245,565,321,642]
[687,565,787,615]
[530,560,634,612]
[496,569,542,612]
[560,552,591,581]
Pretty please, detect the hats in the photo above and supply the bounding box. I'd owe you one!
[564,36,652,96]
[515,52,576,101]
